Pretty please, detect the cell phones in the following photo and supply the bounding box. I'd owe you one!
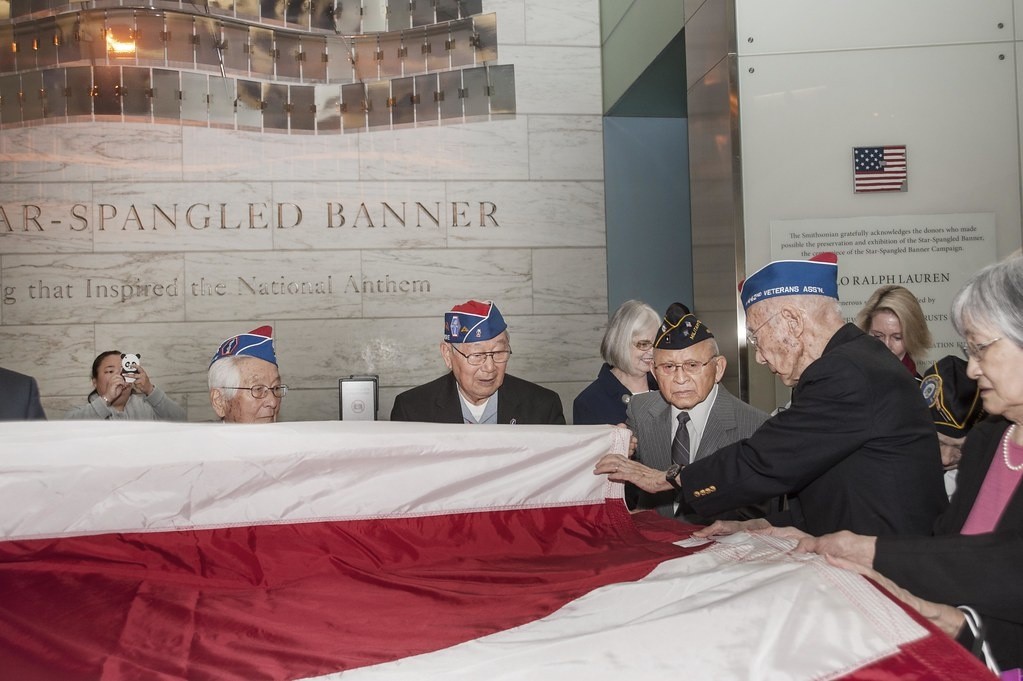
[119,353,142,384]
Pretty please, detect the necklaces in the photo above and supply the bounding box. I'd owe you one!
[1002,424,1023,470]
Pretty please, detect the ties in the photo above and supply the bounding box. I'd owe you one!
[670,411,690,505]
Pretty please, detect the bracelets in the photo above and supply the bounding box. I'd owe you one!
[102,395,109,402]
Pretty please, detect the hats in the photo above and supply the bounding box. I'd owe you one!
[444,300,508,342]
[737,251,839,310]
[207,325,278,368]
[653,301,714,351]
[919,355,989,438]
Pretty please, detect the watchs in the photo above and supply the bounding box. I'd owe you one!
[666,463,684,489]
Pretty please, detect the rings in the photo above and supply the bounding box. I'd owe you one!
[616,465,619,471]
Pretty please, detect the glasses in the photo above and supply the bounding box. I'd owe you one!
[747,311,784,351]
[632,339,654,351]
[448,341,512,366]
[960,332,1014,362]
[219,384,290,399]
[653,354,718,375]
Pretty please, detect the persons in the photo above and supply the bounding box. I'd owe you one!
[593,252,951,537]
[851,283,935,390]
[913,356,990,502]
[390,299,566,425]
[64,349,184,421]
[617,301,772,523]
[572,299,665,511]
[206,326,287,422]
[0,366,46,421]
[788,248,1023,681]
